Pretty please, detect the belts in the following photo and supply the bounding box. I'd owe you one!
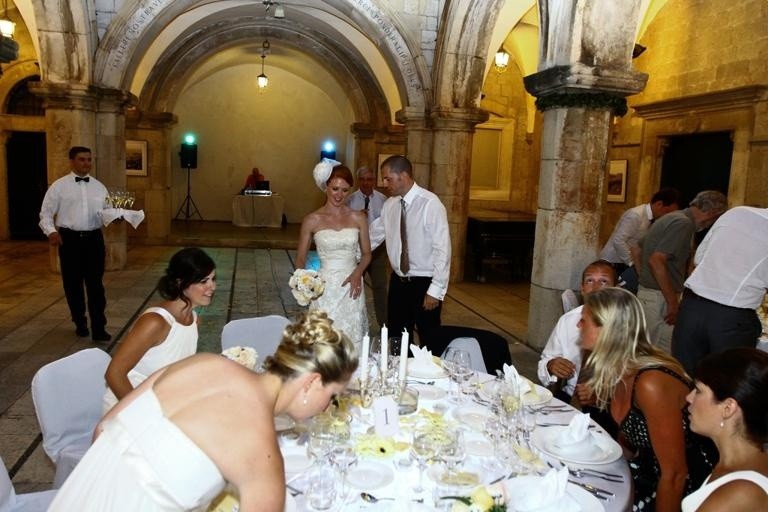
[391,271,434,283]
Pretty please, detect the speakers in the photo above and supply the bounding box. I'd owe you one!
[321,151,335,161]
[181,144,197,168]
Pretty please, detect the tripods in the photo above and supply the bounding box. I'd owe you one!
[173,172,203,222]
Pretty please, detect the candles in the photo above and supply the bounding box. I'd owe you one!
[379,322,389,372]
[399,325,410,382]
[359,330,370,382]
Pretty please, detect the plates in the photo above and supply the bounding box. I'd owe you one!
[272,336,628,512]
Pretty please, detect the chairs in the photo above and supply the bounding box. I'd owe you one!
[30,346,114,469]
[431,323,515,379]
[559,287,579,315]
[0,453,63,512]
[217,313,294,376]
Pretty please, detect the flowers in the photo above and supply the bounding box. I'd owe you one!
[288,267,325,307]
[439,480,508,512]
[219,346,258,372]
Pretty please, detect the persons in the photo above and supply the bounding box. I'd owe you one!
[294,158,372,349]
[346,165,388,331]
[103,247,217,417]
[357,155,452,357]
[39,147,125,341]
[47,310,358,512]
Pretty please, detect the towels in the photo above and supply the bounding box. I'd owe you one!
[521,461,570,512]
[557,410,592,449]
[501,362,534,396]
[407,344,446,379]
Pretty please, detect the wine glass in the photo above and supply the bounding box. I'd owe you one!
[104,191,136,214]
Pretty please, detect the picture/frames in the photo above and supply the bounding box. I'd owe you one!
[375,152,406,190]
[603,157,630,205]
[124,139,149,178]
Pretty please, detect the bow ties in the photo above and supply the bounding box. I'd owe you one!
[74,176,90,183]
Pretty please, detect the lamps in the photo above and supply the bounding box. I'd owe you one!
[0,0,17,42]
[256,51,268,90]
[494,42,511,73]
[260,0,285,19]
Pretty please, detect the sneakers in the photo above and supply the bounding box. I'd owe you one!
[90,327,112,342]
[75,325,90,338]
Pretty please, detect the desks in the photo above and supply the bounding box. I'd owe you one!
[230,193,286,231]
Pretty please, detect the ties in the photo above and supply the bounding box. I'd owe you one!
[364,197,371,211]
[397,197,411,274]
